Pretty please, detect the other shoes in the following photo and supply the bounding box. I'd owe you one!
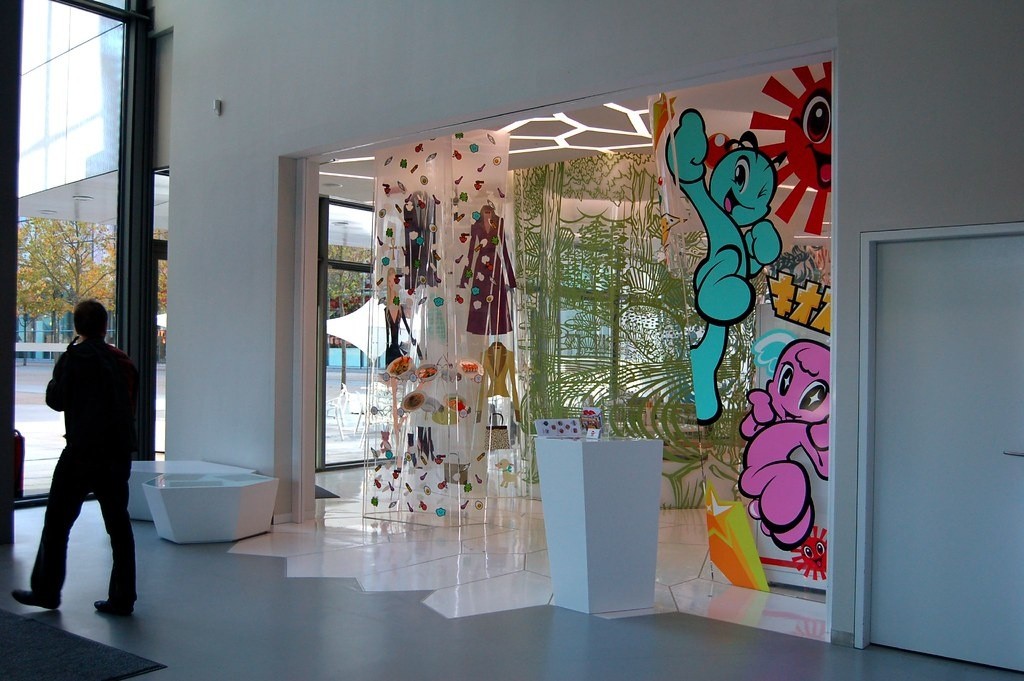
[11,589,61,609]
[94,601,133,617]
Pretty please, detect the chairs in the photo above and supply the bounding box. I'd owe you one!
[325,381,403,450]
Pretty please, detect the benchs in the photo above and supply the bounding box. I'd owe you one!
[128,459,279,545]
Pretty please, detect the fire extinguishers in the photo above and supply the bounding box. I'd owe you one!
[15,429,26,500]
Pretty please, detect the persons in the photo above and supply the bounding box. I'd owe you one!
[11,300,139,615]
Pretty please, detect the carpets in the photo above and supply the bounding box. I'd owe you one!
[0,607,168,681]
